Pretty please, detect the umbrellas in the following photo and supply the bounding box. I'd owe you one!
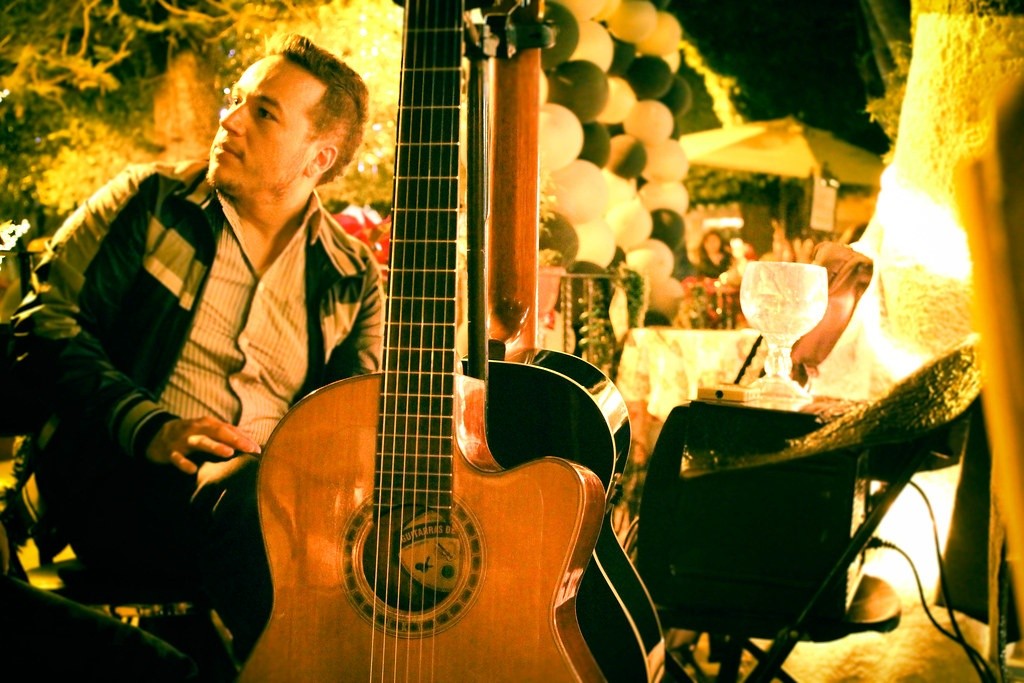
[681,118,885,243]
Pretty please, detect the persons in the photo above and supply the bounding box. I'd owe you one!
[0,31,384,664]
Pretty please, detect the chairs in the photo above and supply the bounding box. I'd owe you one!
[635,473,915,683]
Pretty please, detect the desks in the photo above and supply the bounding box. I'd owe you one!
[628,325,769,399]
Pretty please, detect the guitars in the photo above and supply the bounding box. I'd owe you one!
[232,0,609,683]
[460,0,667,683]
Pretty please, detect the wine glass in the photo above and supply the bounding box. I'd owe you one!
[741,263,827,399]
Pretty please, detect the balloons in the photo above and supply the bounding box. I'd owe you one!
[538,0,698,379]
